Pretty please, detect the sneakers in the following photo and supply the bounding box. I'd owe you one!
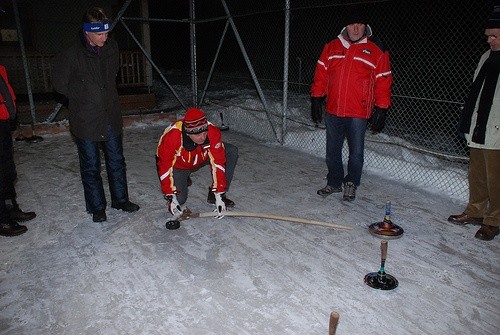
[342,181,357,201]
[317,184,343,197]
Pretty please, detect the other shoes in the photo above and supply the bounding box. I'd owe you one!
[93,209,106,222]
[187,176,192,186]
[207,187,235,207]
[111,201,140,213]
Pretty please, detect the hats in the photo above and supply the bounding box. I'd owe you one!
[183,108,208,134]
[485,7,500,29]
[342,7,368,27]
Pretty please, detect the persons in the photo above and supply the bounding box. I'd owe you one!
[53,7,139,223]
[309,8,393,200]
[0,65,37,237]
[448,11,500,240]
[156,107,239,219]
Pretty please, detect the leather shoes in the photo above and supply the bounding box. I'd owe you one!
[474,224,500,241]
[0,207,36,237]
[448,212,484,226]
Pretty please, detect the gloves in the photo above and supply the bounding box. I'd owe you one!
[367,105,388,135]
[310,96,325,124]
[168,194,183,216]
[211,191,228,220]
